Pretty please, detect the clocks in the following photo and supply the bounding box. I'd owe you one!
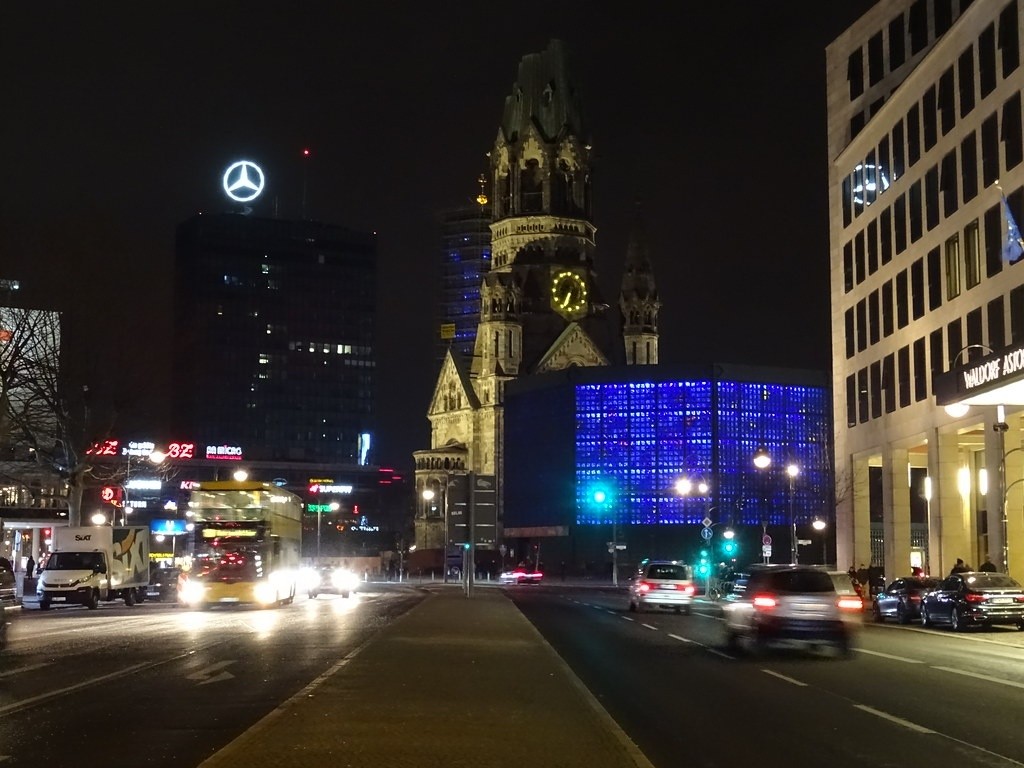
[551,272,587,312]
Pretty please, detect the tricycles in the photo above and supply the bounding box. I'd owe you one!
[709,572,750,602]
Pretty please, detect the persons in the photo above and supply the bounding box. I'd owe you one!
[980,554,997,572]
[950,558,969,574]
[26,556,35,578]
[857,564,868,598]
[848,566,857,587]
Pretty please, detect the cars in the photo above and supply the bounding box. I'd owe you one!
[872,577,944,624]
[0,556,18,607]
[920,572,1024,633]
[501,568,543,585]
[139,567,183,602]
[306,566,353,598]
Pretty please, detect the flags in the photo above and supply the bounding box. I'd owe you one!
[1000,190,1023,261]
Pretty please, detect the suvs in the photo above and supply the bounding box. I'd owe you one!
[629,558,697,616]
[722,563,845,662]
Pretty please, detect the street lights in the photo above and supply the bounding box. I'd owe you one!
[591,486,618,585]
[423,468,449,583]
[788,465,800,565]
[753,451,773,565]
[813,515,827,565]
[676,473,712,599]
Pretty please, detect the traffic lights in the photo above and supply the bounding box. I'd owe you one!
[101,487,123,506]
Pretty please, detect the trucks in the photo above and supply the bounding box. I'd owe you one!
[37,526,152,610]
[177,480,304,610]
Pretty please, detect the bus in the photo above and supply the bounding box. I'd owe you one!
[1,521,68,574]
[149,518,190,566]
